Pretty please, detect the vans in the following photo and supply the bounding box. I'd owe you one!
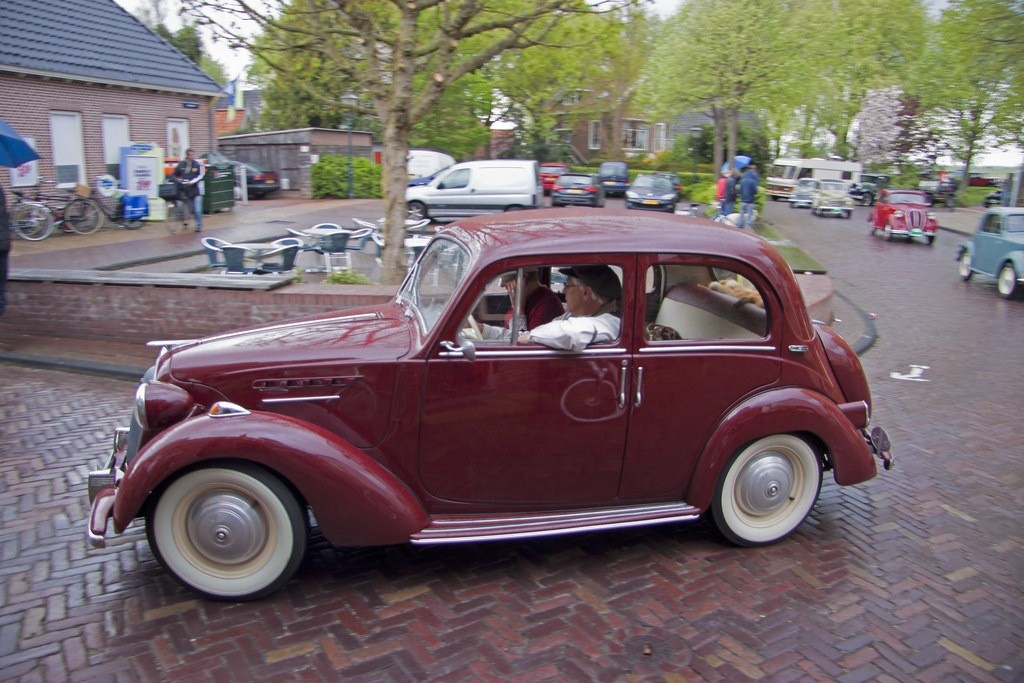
[403,159,544,224]
[598,162,630,196]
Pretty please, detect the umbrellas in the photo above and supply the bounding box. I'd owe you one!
[720,155,752,173]
[0,119,45,168]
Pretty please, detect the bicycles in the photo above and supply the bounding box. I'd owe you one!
[159,177,191,232]
[8,177,149,241]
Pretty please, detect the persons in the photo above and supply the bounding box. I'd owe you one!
[172,148,207,231]
[463,262,622,352]
[716,168,759,228]
[0,185,15,315]
[497,266,565,331]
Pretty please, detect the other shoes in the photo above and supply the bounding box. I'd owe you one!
[194,228,201,232]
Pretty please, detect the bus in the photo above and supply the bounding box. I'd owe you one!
[764,158,863,200]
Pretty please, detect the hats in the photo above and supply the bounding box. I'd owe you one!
[500,267,538,288]
[558,264,623,303]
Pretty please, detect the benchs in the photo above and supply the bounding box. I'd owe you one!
[655,284,767,340]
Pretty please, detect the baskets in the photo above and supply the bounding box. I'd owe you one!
[158,184,180,202]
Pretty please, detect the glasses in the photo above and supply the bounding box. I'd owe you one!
[562,282,582,291]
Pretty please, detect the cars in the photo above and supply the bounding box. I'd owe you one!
[789,173,895,215]
[954,208,1024,298]
[917,168,1002,211]
[88,206,894,604]
[867,189,938,245]
[230,160,281,198]
[626,171,682,213]
[539,162,568,196]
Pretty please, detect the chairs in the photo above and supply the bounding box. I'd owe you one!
[201,216,445,278]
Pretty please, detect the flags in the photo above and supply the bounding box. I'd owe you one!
[224,79,244,122]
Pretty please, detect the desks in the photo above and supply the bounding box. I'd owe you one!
[377,220,417,225]
[403,239,430,247]
[302,228,353,235]
[223,243,285,250]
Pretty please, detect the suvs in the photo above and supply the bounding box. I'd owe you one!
[550,172,607,208]
